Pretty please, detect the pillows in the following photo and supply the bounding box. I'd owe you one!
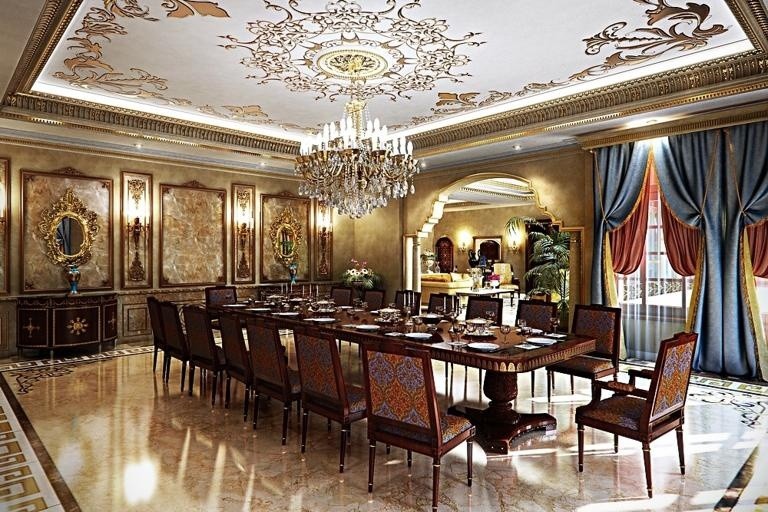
[450,272,463,282]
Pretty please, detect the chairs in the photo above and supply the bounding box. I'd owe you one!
[574,332,698,498]
[466,263,521,298]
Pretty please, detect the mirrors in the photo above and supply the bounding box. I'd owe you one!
[474,236,502,264]
[270,202,303,270]
[38,187,100,268]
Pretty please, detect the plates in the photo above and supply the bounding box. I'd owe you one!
[221,299,567,351]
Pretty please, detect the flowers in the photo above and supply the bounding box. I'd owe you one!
[343,258,384,289]
[420,250,435,260]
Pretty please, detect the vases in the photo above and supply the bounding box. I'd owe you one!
[423,260,433,274]
[468,249,477,269]
[351,281,363,288]
[67,265,80,295]
[289,263,297,284]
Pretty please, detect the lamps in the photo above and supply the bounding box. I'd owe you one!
[295,61,419,219]
[507,230,522,255]
[457,232,471,256]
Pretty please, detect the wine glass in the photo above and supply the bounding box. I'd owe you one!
[434,261,440,273]
[483,282,493,290]
[246,294,560,347]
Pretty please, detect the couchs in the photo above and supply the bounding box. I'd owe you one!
[422,272,472,309]
